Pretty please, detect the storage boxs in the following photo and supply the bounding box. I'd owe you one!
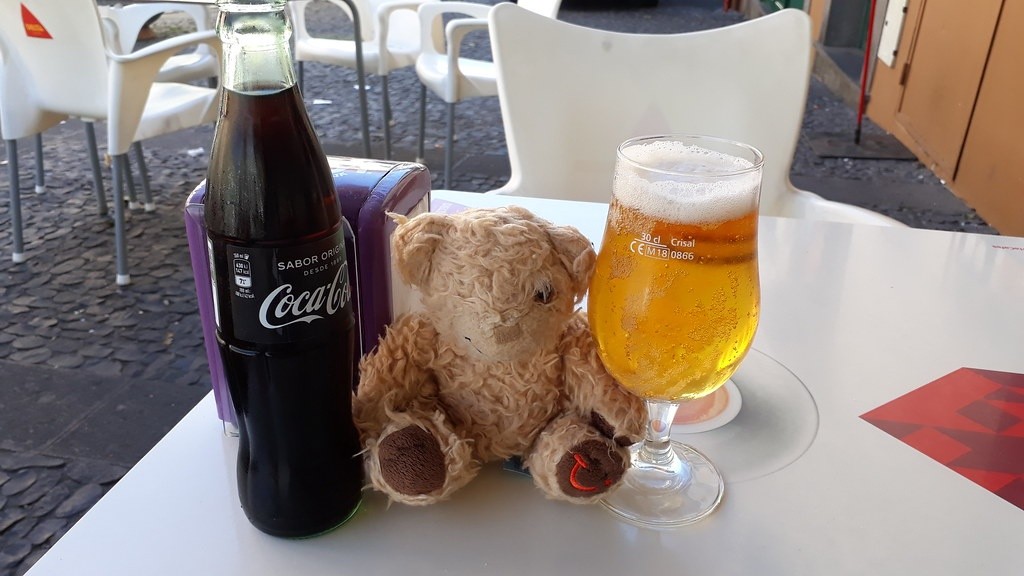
[184,154,431,430]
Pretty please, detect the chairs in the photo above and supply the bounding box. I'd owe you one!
[483,2,914,230]
[0,0,222,286]
[287,0,457,161]
[415,0,563,191]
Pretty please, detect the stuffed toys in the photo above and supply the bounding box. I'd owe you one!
[352,205,647,507]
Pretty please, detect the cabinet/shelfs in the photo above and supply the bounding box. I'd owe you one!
[895,0,1023,238]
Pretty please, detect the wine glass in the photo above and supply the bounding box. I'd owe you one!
[585,134,765,527]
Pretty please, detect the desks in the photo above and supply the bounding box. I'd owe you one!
[21,189,1024,575]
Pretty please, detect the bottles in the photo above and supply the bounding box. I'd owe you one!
[202,0,368,541]
